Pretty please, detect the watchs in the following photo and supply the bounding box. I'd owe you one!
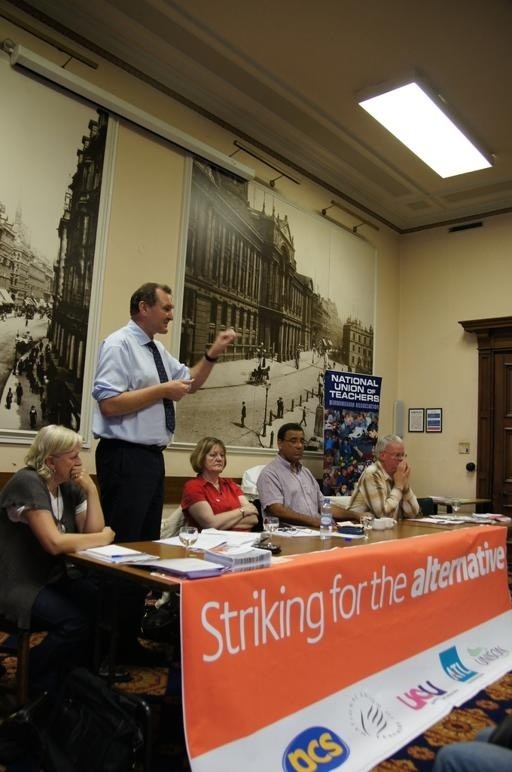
[240,506,246,518]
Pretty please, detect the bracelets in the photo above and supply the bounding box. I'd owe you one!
[204,350,218,362]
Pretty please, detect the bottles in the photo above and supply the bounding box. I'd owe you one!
[319,497,334,540]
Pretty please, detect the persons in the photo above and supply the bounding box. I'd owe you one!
[241,402,247,425]
[181,436,259,533]
[344,434,420,518]
[323,409,377,496]
[0,423,116,677]
[256,423,374,528]
[277,398,284,418]
[299,406,308,426]
[91,282,236,681]
[0,296,51,430]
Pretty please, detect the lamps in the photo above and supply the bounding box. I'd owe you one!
[353,71,498,181]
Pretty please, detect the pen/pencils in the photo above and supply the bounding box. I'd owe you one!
[112,553,142,558]
[278,528,297,531]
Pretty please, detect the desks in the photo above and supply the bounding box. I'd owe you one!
[66,518,511,771]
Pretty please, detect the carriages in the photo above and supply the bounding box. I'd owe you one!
[249,364,270,387]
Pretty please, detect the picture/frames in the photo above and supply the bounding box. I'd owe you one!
[166,156,380,459]
[0,48,122,449]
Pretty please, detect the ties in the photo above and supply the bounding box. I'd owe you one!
[147,341,176,435]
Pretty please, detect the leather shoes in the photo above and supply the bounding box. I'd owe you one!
[97,662,132,684]
[129,645,168,668]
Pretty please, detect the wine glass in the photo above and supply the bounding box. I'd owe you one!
[451,499,462,517]
[179,525,200,558]
[263,515,280,547]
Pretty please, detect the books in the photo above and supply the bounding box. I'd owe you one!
[409,512,511,527]
[77,530,272,582]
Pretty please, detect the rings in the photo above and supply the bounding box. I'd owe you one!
[76,474,77,477]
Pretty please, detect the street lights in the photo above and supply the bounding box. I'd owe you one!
[11,328,22,377]
[260,380,271,438]
[255,338,268,369]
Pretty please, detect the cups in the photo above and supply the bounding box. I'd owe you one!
[380,516,399,531]
[360,511,375,530]
[371,519,387,530]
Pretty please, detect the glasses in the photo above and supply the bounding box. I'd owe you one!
[282,438,306,443]
[382,449,407,457]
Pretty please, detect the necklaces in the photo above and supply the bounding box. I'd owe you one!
[57,486,66,533]
